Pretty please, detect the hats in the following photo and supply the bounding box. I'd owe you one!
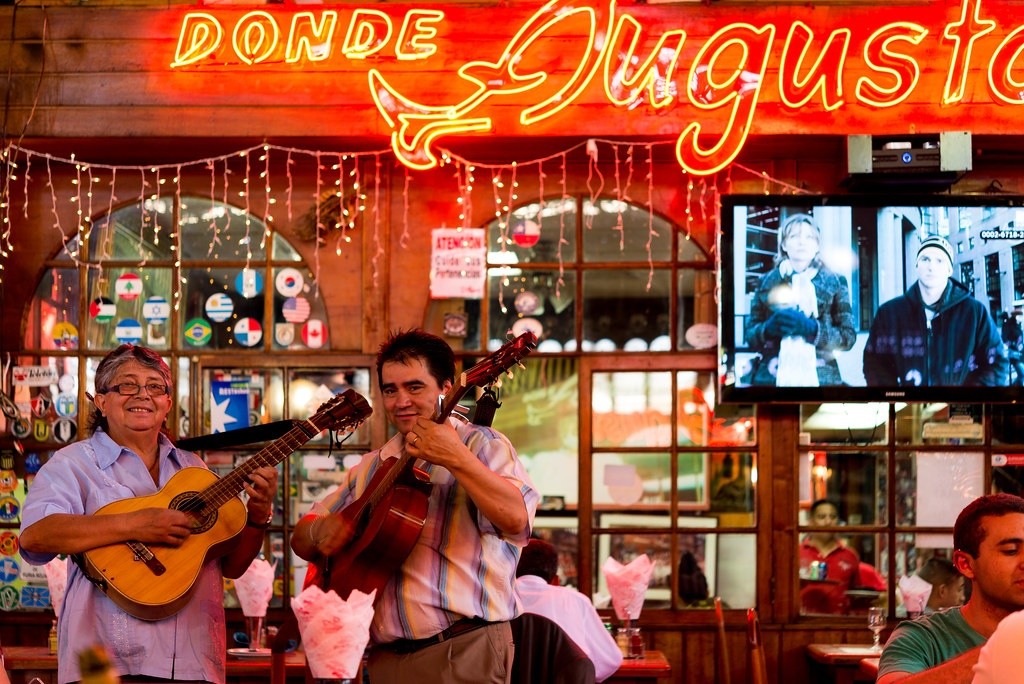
[917,236,953,264]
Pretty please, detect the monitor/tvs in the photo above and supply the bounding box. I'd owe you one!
[718,193,1024,402]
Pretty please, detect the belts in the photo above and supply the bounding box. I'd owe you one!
[386,616,499,654]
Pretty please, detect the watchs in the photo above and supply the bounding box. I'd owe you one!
[248,512,273,528]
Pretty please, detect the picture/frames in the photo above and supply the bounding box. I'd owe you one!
[598,511,720,603]
[475,357,712,512]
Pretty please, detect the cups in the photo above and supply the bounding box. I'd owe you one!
[618,618,639,629]
[245,616,264,652]
[906,611,924,621]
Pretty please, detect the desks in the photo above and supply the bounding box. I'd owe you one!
[858,657,882,679]
[808,642,884,684]
[613,649,671,684]
[4,646,307,679]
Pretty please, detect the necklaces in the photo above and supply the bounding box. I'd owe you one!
[149,446,158,473]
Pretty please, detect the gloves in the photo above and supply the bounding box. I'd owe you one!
[764,307,818,344]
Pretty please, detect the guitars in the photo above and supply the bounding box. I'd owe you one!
[71,389,373,621]
[302,331,538,608]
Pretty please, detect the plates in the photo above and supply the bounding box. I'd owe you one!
[227,647,271,657]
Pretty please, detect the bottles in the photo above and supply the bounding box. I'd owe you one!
[48,620,58,655]
[616,628,628,658]
[628,628,645,658]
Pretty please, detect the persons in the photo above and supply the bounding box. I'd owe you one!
[863,237,1009,386]
[515,539,622,684]
[747,213,858,385]
[797,500,886,613]
[290,333,535,684]
[921,557,965,609]
[875,492,1024,684]
[18,345,279,684]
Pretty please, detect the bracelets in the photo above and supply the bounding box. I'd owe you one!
[310,516,323,540]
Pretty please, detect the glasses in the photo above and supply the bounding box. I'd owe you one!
[100,383,168,396]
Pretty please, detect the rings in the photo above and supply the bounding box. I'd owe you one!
[413,436,418,444]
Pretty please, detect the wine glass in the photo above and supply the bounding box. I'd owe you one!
[868,607,886,652]
